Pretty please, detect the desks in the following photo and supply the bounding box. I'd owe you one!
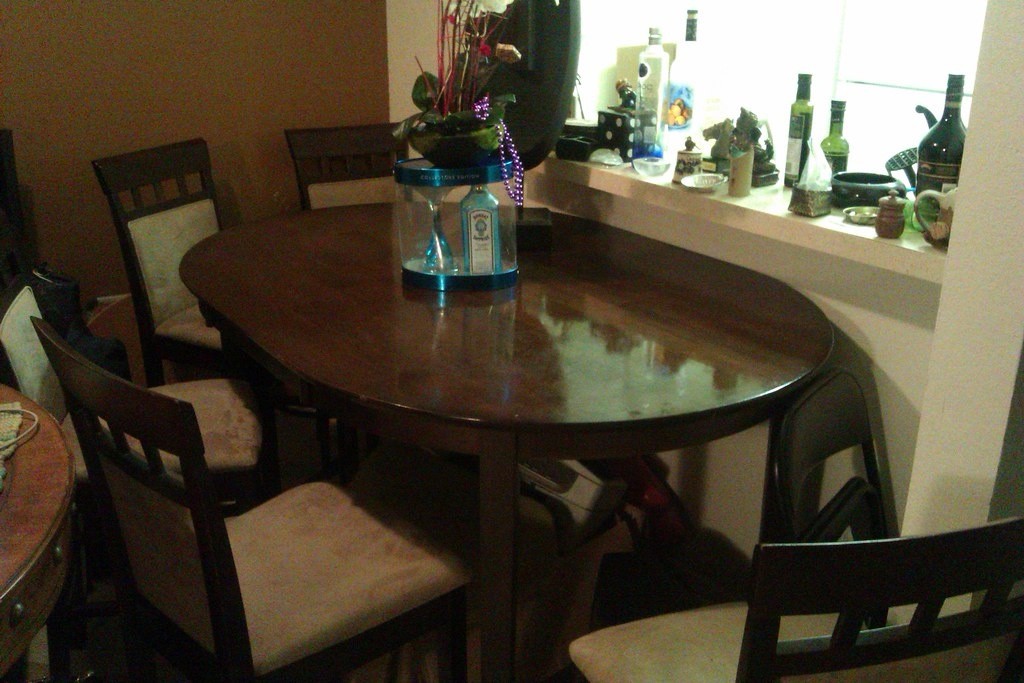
[0,383,76,683]
[179,205,832,682]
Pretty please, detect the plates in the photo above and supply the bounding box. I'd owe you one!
[843,205,879,224]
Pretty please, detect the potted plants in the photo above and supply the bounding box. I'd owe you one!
[391,0,524,162]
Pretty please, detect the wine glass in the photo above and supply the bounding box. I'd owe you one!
[412,185,459,275]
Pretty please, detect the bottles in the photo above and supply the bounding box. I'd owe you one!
[915,72,967,210]
[631,29,671,171]
[820,98,850,177]
[874,189,907,239]
[461,182,502,275]
[665,9,703,144]
[784,74,814,189]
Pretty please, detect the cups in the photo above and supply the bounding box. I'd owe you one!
[913,188,957,250]
[672,140,703,184]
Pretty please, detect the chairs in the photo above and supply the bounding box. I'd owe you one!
[0,121,1024,683]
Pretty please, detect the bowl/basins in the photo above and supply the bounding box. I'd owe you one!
[678,173,728,193]
[831,171,906,208]
[633,157,670,178]
